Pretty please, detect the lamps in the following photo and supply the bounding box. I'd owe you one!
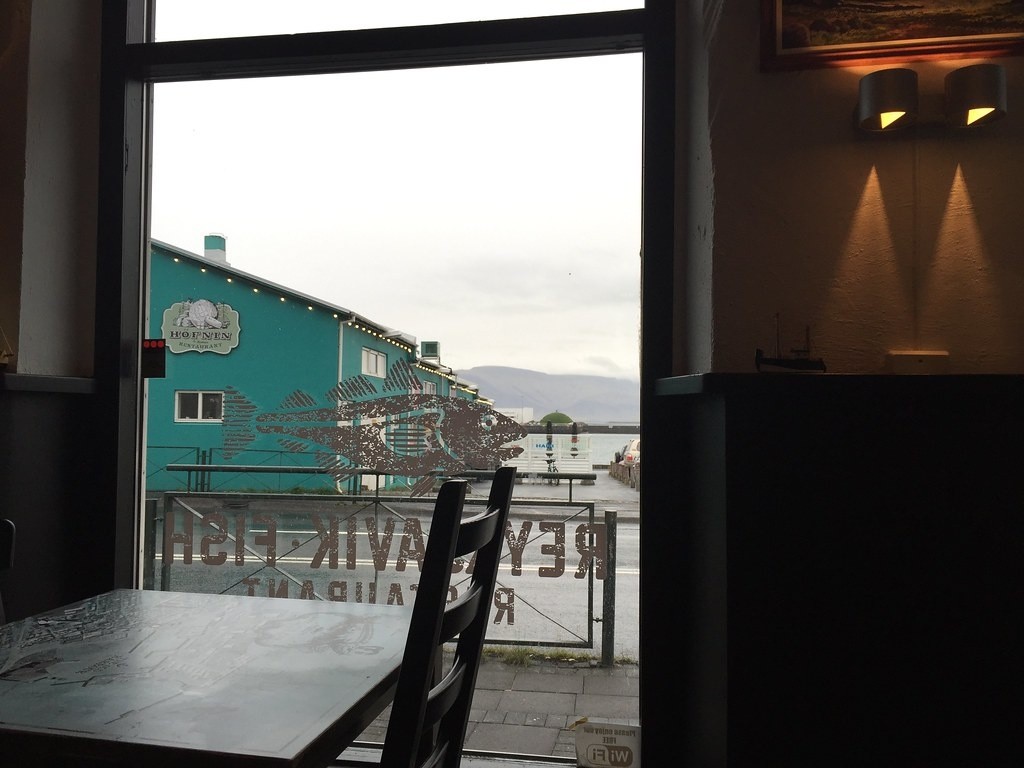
[855,68,920,133]
[945,63,1008,129]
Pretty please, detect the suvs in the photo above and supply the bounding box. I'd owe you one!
[618,439,640,468]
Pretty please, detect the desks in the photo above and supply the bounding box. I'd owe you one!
[0,586,415,768]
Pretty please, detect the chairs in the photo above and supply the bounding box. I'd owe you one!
[340,466,518,768]
